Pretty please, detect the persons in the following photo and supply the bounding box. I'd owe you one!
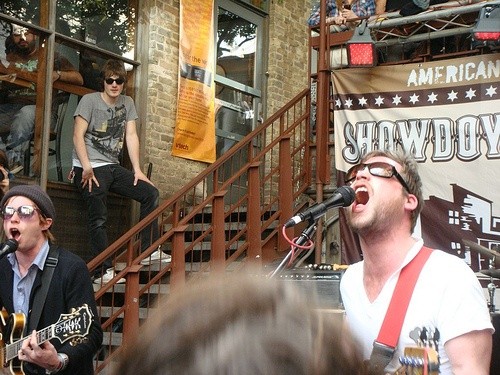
[69,58,172,284]
[0,24,84,174]
[0,186,104,375]
[308,0,378,30]
[338,151,495,375]
[115,260,374,375]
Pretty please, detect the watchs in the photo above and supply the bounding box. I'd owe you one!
[56,69,61,82]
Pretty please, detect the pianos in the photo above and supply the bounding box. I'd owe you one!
[274,270,345,310]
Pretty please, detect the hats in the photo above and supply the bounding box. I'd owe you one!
[0,183,55,220]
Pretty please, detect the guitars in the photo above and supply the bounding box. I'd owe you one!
[0,304,94,375]
[363,346,439,374]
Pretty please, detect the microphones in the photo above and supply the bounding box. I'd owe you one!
[0,239,19,259]
[283,186,355,228]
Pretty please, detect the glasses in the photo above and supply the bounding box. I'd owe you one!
[104,76,125,85]
[0,205,43,223]
[344,162,412,194]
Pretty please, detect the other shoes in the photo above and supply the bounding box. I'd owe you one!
[139,249,173,265]
[94,268,127,284]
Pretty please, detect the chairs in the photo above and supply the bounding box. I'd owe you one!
[0,88,71,183]
